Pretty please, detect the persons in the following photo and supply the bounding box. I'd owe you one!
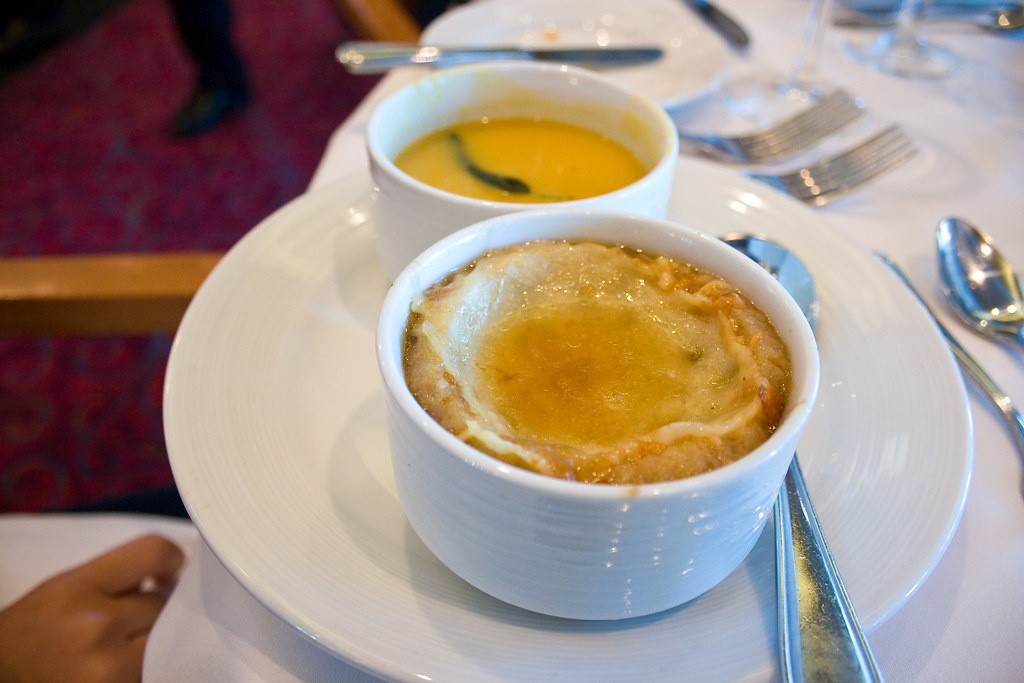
[0,512,368,683]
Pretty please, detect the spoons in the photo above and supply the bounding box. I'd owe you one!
[923,9,1024,29]
[718,232,886,683]
[935,216,1024,358]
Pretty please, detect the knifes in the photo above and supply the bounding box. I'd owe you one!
[335,47,664,74]
[875,251,1024,452]
[685,0,747,45]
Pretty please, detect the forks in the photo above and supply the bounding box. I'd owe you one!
[678,91,917,208]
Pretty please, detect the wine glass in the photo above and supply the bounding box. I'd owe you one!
[843,0,965,80]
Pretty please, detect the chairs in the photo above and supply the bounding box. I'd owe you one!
[0,253,229,683]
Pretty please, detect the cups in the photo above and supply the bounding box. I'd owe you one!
[365,61,680,279]
[373,210,821,621]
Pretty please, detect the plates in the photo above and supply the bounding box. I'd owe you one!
[162,151,974,683]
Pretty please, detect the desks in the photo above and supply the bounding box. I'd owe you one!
[139,0,1024,683]
